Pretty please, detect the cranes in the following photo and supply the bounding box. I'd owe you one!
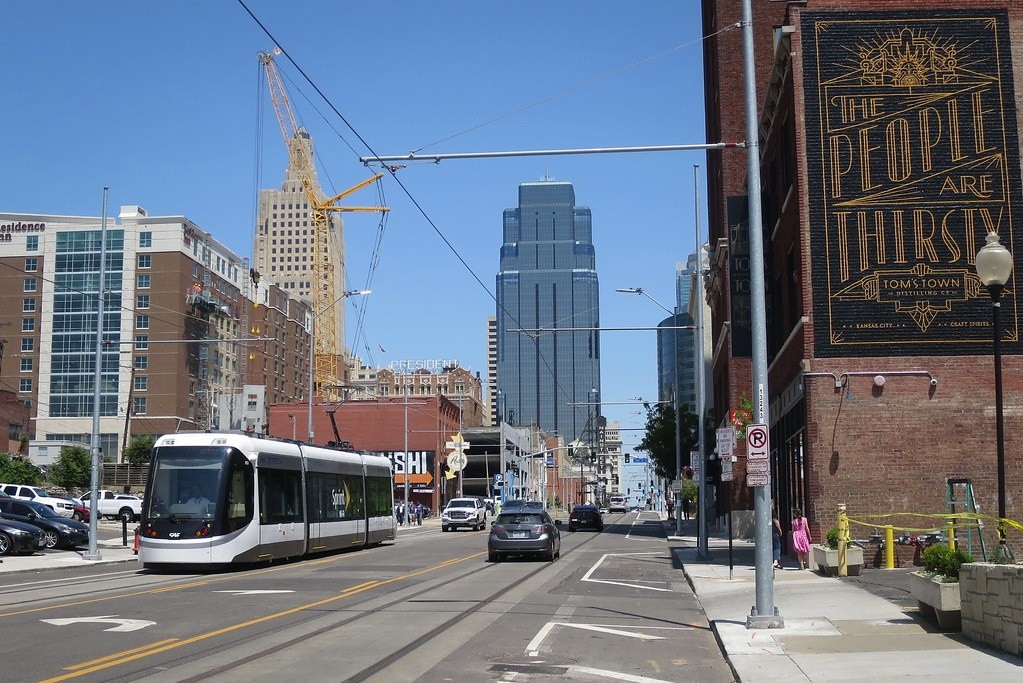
[260,52,390,402]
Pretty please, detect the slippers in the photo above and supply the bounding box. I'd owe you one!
[773,564,783,570]
[799,567,805,570]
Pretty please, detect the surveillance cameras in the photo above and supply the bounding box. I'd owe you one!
[930,379,937,386]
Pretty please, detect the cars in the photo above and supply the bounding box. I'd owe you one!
[0,500,89,550]
[499,500,543,513]
[0,516,47,557]
[599,506,640,513]
[395,500,431,518]
[569,506,604,533]
[106,494,140,519]
[48,492,103,522]
[486,509,563,562]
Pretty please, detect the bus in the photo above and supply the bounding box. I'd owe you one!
[138,431,398,568]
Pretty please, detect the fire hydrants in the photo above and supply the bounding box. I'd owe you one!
[132,526,141,554]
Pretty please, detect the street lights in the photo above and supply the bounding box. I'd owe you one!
[307,288,373,445]
[460,379,494,497]
[614,288,682,536]
[628,396,665,513]
[519,429,559,499]
[976,229,1013,563]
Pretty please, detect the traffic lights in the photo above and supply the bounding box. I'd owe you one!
[625,453,630,463]
[638,483,641,489]
[592,452,596,462]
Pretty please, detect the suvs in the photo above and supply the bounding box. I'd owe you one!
[0,483,74,518]
[609,497,627,513]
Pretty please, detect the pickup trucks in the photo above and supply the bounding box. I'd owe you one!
[483,495,501,507]
[71,490,144,522]
[440,496,487,532]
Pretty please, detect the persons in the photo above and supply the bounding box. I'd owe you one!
[495,502,500,516]
[681,499,689,520]
[486,501,491,516]
[193,283,202,293]
[666,498,673,520]
[409,501,423,526]
[397,501,404,526]
[791,508,812,570]
[771,501,783,570]
[187,485,210,512]
